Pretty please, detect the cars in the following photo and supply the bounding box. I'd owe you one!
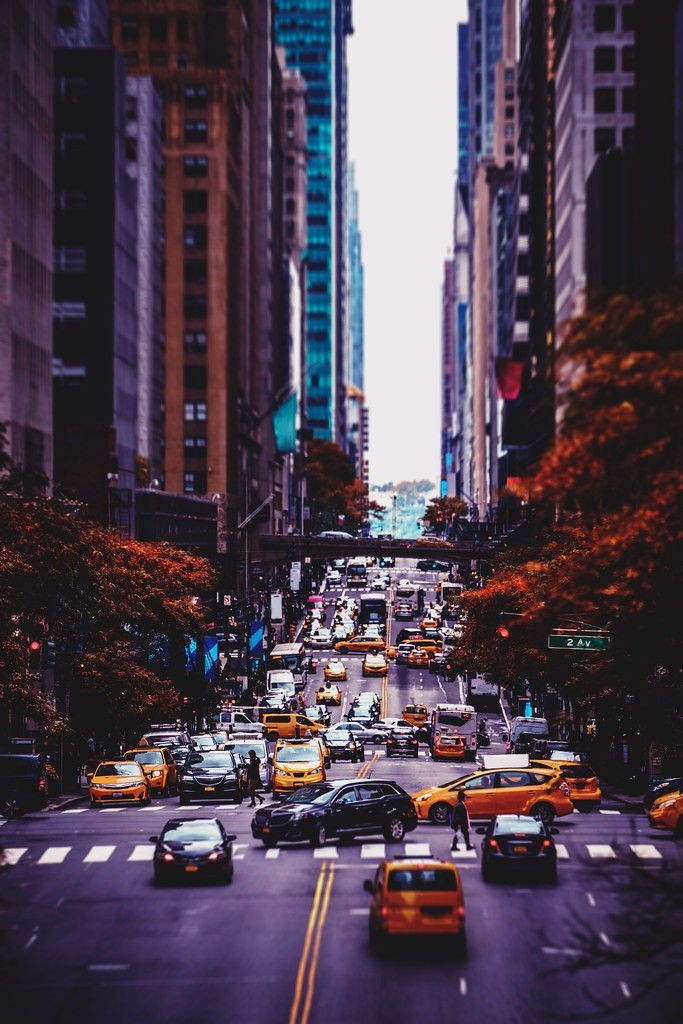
[148,817,237,887]
[416,535,456,549]
[643,776,683,838]
[431,735,467,763]
[410,767,575,826]
[476,759,602,815]
[86,759,152,808]
[363,855,468,957]
[475,814,560,881]
[319,531,355,541]
[139,557,591,789]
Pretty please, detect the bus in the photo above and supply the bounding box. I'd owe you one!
[426,704,479,762]
[268,645,306,692]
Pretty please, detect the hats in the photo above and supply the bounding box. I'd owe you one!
[457,792,467,800]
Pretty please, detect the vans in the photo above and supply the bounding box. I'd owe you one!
[502,716,549,754]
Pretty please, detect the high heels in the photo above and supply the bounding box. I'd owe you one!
[260,797,265,804]
[247,802,256,807]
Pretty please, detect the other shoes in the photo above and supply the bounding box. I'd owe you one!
[451,847,460,851]
[467,846,475,850]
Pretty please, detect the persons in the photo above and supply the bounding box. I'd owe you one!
[450,792,476,850]
[245,750,266,808]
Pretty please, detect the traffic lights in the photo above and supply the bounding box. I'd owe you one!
[496,622,512,643]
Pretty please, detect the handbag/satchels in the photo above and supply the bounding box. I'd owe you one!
[449,813,455,829]
[455,828,463,840]
[255,779,263,789]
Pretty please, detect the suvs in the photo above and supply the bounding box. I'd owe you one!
[383,728,421,759]
[121,746,179,798]
[0,752,50,821]
[251,778,419,848]
[174,749,247,806]
